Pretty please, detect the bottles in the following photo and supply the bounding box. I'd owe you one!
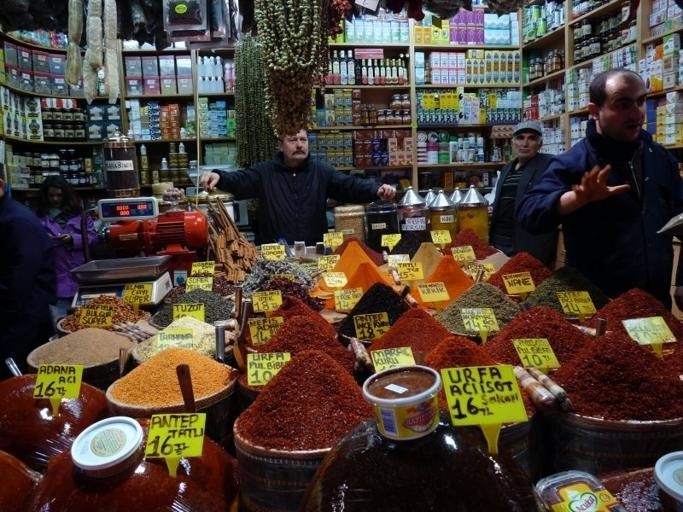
[522,5,565,44]
[433,92,440,110]
[449,132,511,164]
[361,93,411,126]
[102,126,140,189]
[198,56,236,94]
[523,48,565,84]
[325,49,409,85]
[334,185,488,250]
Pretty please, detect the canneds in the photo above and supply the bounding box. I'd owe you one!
[523,0,638,82]
[24,107,98,187]
[352,88,412,127]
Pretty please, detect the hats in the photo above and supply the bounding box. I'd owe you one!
[512,121,541,136]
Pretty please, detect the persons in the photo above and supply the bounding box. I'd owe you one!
[0,162,58,382]
[199,123,397,247]
[513,66,683,314]
[488,118,559,271]
[33,174,99,331]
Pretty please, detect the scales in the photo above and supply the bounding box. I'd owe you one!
[70,255,173,309]
